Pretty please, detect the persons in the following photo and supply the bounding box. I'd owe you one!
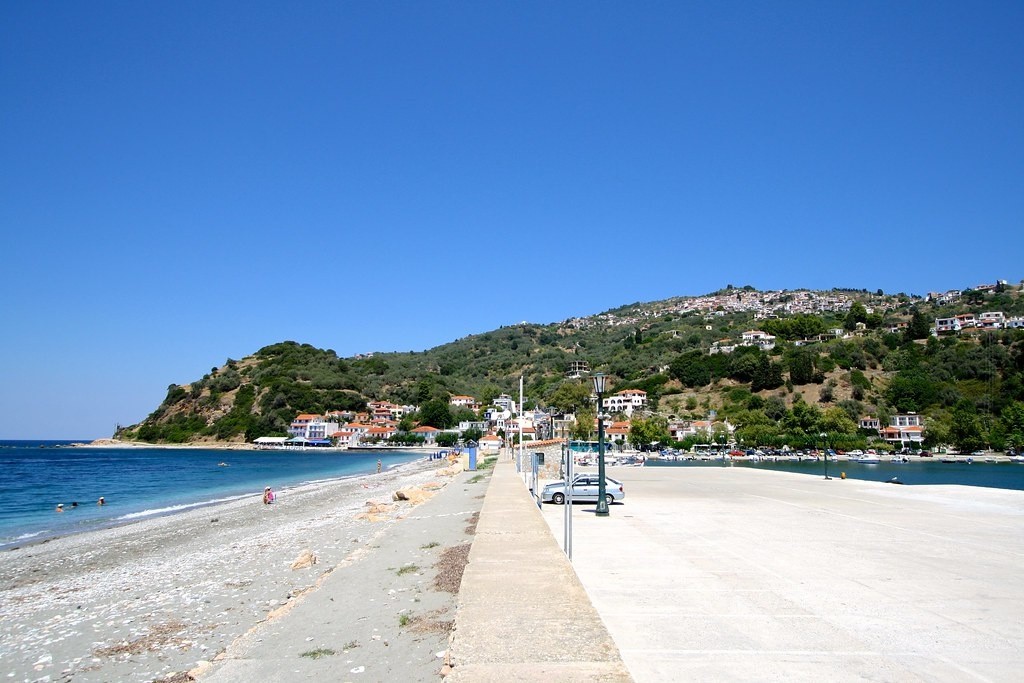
[218,462,224,466]
[448,450,460,456]
[264,486,274,505]
[56,497,104,512]
[377,460,381,473]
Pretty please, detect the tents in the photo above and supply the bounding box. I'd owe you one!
[440,450,448,457]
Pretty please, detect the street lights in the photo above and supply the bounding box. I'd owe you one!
[592,372,610,517]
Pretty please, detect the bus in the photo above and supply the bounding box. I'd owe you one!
[570,440,613,452]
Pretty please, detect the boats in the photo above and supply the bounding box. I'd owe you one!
[857,454,1024,464]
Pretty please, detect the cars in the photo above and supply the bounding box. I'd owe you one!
[541,473,625,505]
[660,448,875,457]
